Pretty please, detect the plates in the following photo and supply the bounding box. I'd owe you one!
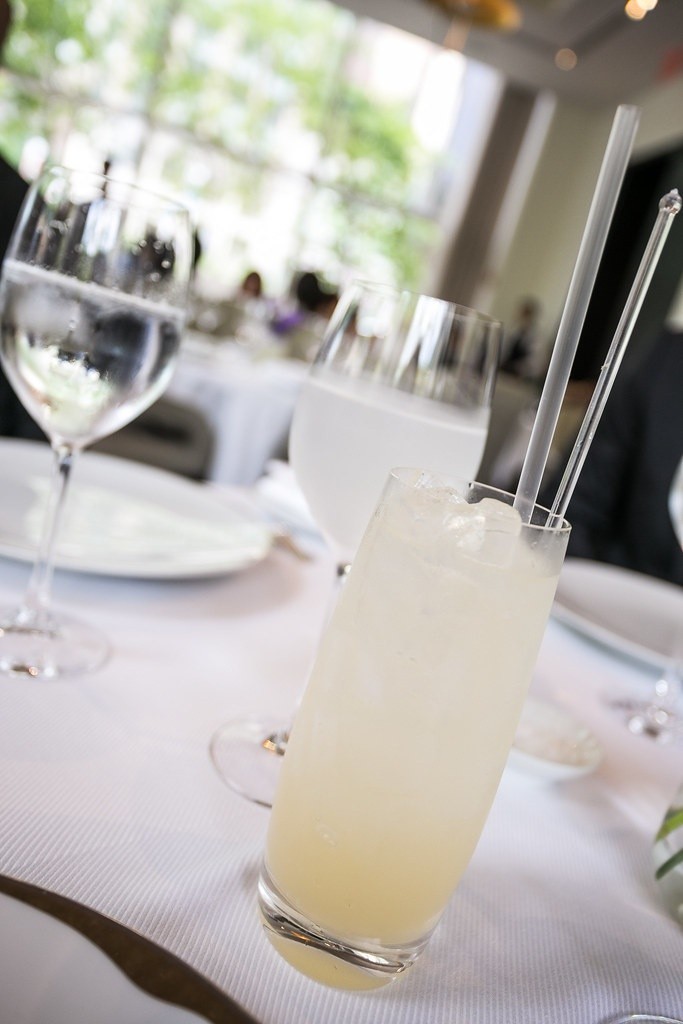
[548,554,683,674]
[502,672,605,784]
[0,435,266,580]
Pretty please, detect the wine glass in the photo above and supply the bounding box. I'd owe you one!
[206,278,502,810]
[0,160,197,682]
[603,452,683,748]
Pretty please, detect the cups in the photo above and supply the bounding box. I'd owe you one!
[258,465,571,993]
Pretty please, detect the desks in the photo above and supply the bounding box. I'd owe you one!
[0,258,313,485]
[0,532,683,1024]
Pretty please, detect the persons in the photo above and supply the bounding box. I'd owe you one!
[243,270,262,299]
[269,271,357,343]
